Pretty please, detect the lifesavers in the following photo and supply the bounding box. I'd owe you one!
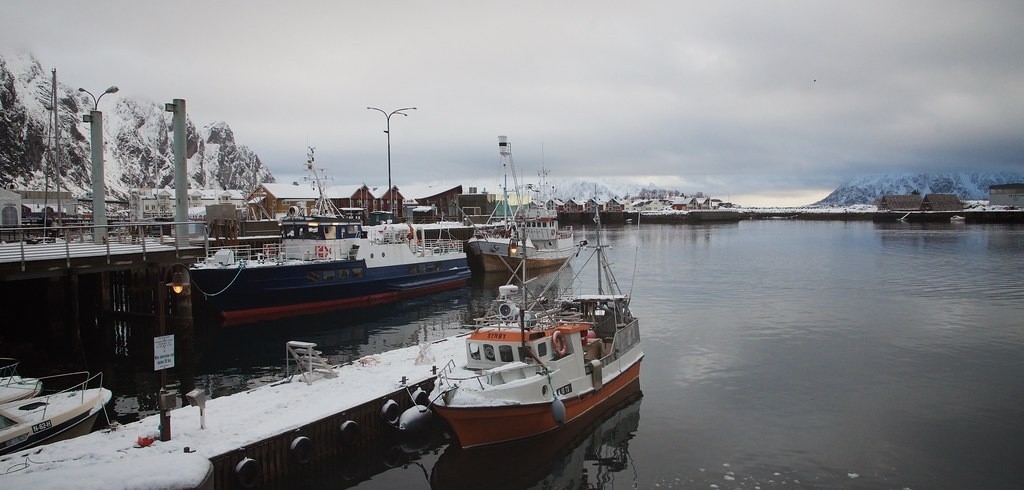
[234,457,260,488]
[411,389,428,407]
[552,330,568,356]
[551,229,555,235]
[265,245,275,254]
[381,401,400,425]
[340,420,360,448]
[320,246,328,257]
[290,436,313,465]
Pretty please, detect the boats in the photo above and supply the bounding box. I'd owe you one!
[429,138,643,455]
[949,214,964,223]
[0,357,113,455]
[164,135,581,340]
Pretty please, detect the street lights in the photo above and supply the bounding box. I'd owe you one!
[367,105,419,213]
[78,85,120,245]
